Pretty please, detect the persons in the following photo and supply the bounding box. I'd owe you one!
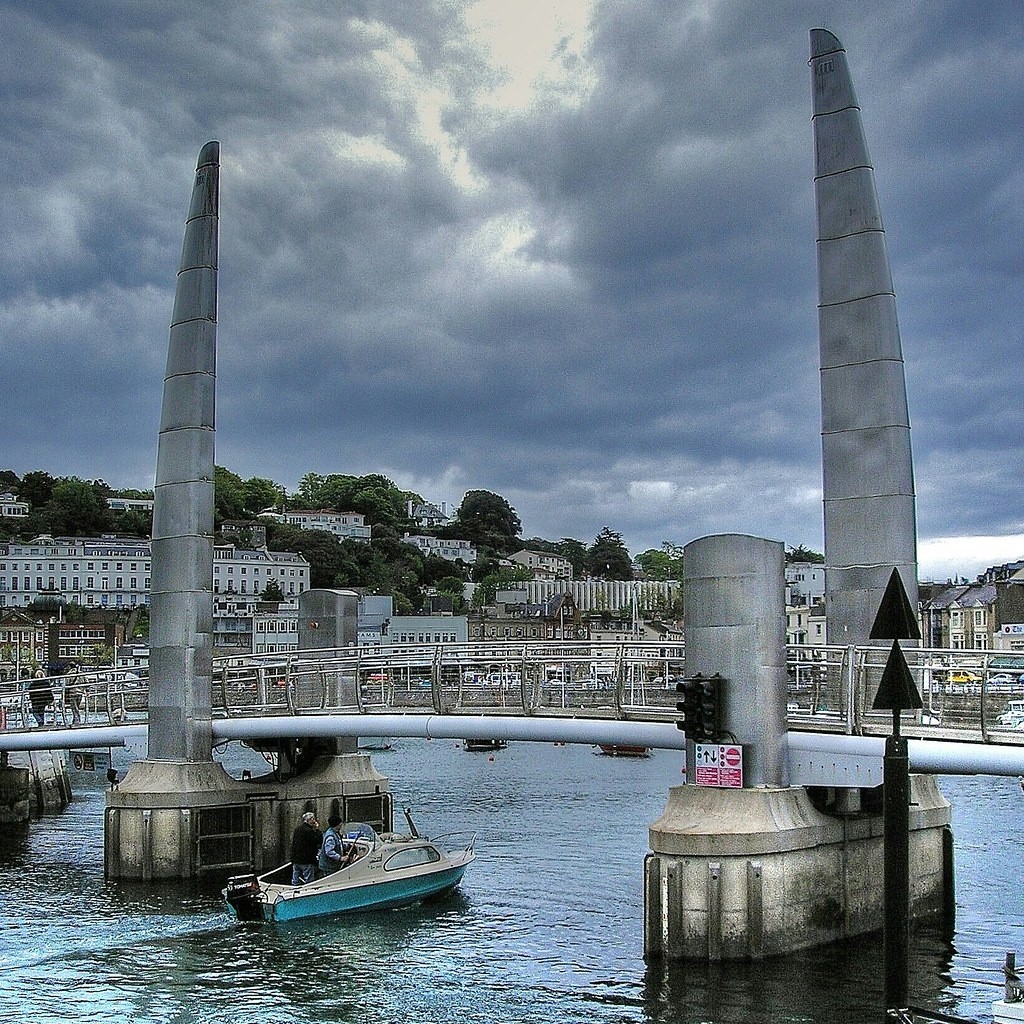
[65,662,90,726]
[28,670,54,727]
[290,812,323,885]
[1010,712,1024,728]
[319,815,349,876]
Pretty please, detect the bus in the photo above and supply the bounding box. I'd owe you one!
[464,671,521,686]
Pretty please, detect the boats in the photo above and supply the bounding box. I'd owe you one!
[597,744,649,757]
[219,820,478,924]
[464,738,507,750]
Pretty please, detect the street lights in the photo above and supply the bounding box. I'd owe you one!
[79,624,84,662]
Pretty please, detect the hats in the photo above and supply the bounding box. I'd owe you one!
[328,815,342,828]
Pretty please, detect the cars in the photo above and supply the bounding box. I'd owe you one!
[1020,675,1024,683]
[986,674,1013,684]
[654,677,664,683]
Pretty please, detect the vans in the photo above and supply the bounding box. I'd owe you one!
[946,670,982,684]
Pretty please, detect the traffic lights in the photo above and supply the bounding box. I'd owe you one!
[675,674,723,747]
